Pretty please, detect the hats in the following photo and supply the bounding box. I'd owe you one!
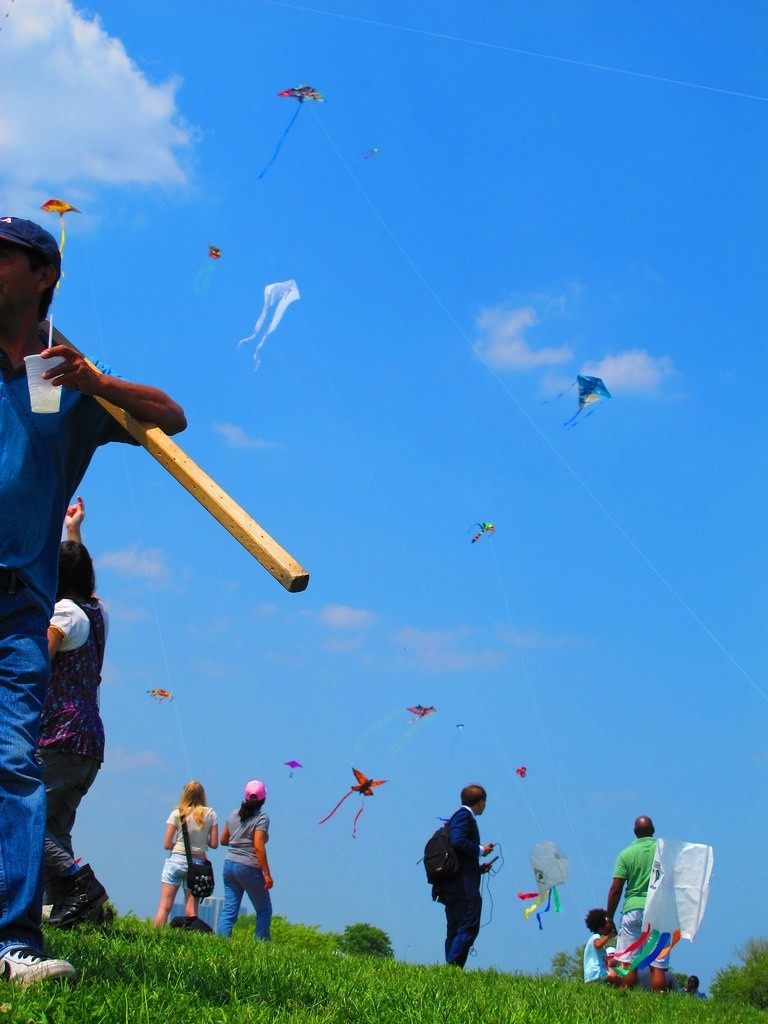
[606,947,616,953]
[0,217,61,279]
[245,780,266,801]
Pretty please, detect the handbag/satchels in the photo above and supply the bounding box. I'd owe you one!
[170,917,213,932]
[188,861,216,898]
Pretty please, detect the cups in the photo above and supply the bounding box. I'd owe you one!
[23,354,65,414]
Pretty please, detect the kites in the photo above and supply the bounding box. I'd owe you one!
[318,767,388,837]
[39,199,80,258]
[257,85,329,179]
[517,842,569,932]
[147,688,175,703]
[236,279,301,371]
[283,760,304,777]
[604,837,714,975]
[469,521,496,543]
[400,703,536,783]
[207,243,223,259]
[363,148,381,158]
[538,375,612,430]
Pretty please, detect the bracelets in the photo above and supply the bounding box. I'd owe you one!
[606,918,613,922]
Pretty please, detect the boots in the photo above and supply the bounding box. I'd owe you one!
[49,891,68,918]
[47,863,109,927]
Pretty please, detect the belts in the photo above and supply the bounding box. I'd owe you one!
[0,569,27,588]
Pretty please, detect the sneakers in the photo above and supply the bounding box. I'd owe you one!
[0,949,77,987]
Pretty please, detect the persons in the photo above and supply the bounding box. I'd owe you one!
[583,816,678,991]
[425,786,494,974]
[220,780,274,941]
[0,217,187,994]
[682,975,710,999]
[154,780,219,930]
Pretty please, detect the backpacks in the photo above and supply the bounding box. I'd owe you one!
[424,809,471,886]
